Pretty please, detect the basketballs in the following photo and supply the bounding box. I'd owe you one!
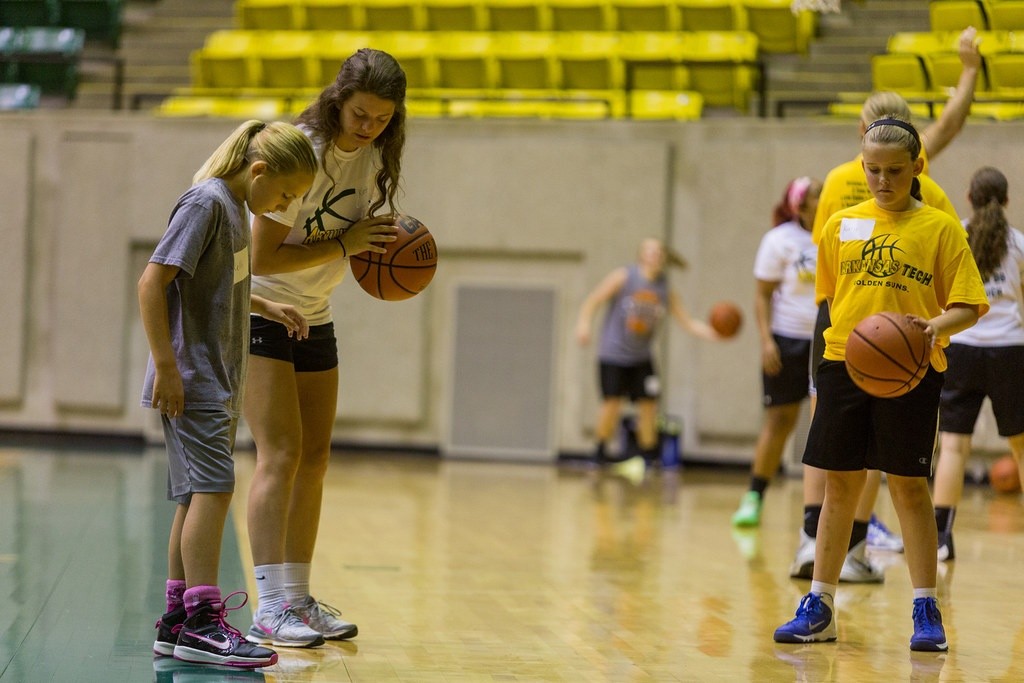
[710,302,741,336]
[845,311,930,398]
[350,214,437,302]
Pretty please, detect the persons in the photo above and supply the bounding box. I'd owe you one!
[775,113,989,652]
[137,120,319,667]
[731,26,1024,583]
[577,237,734,468]
[242,48,405,648]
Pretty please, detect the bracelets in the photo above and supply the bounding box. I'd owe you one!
[335,236,346,257]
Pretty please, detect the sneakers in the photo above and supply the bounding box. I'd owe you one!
[154,609,186,657]
[174,590,278,667]
[842,540,885,584]
[246,603,324,649]
[773,592,837,644]
[910,651,947,683]
[293,596,357,640]
[774,638,838,683]
[152,649,265,683]
[909,597,947,651]
[790,531,819,577]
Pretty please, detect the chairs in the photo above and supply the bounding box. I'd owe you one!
[828,0,1024,121]
[153,0,816,116]
[0,0,133,111]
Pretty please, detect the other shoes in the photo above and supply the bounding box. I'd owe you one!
[733,491,762,525]
[936,534,955,562]
[867,515,906,553]
